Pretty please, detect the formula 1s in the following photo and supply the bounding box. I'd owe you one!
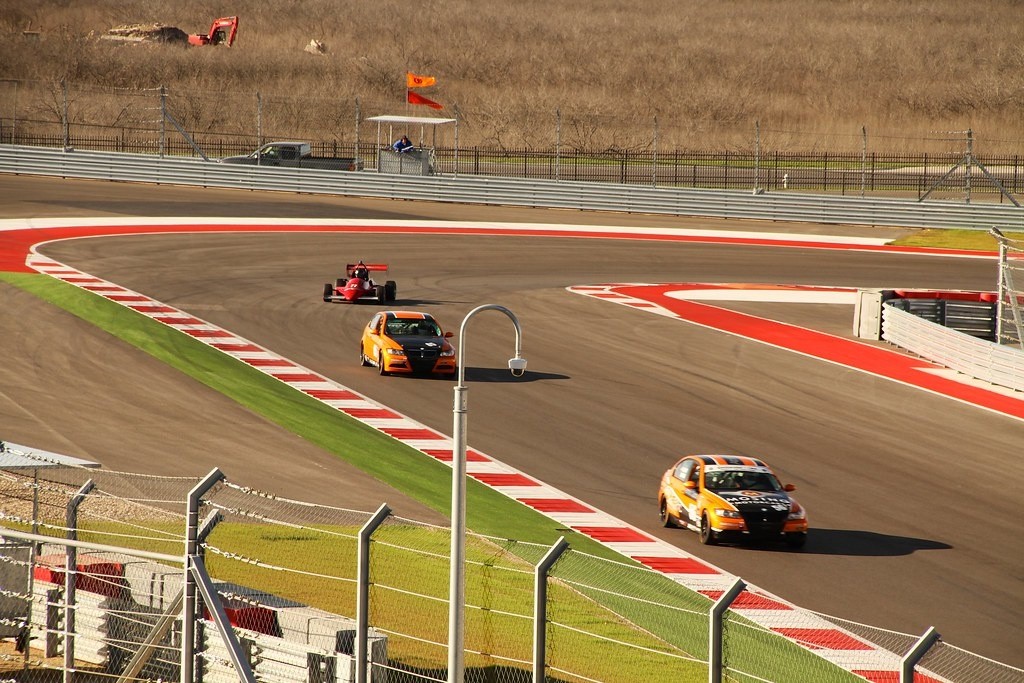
[322,260,398,306]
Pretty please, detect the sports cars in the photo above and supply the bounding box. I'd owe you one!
[656,454,808,550]
[359,311,458,378]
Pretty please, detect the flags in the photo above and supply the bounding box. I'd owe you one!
[408,91,443,110]
[407,72,436,88]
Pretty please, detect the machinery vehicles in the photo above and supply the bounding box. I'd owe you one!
[188,15,240,48]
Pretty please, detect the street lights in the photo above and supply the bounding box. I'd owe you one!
[446,303,545,683]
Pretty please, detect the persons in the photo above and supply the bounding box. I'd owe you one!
[418,321,429,334]
[393,135,413,154]
[738,472,758,488]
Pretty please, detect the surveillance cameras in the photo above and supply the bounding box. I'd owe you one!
[507,356,527,378]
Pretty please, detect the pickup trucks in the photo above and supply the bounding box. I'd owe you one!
[219,140,365,173]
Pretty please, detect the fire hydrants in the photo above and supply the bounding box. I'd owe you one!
[782,173,793,188]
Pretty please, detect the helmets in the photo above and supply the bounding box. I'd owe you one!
[743,473,757,486]
[418,321,430,334]
[355,269,367,280]
